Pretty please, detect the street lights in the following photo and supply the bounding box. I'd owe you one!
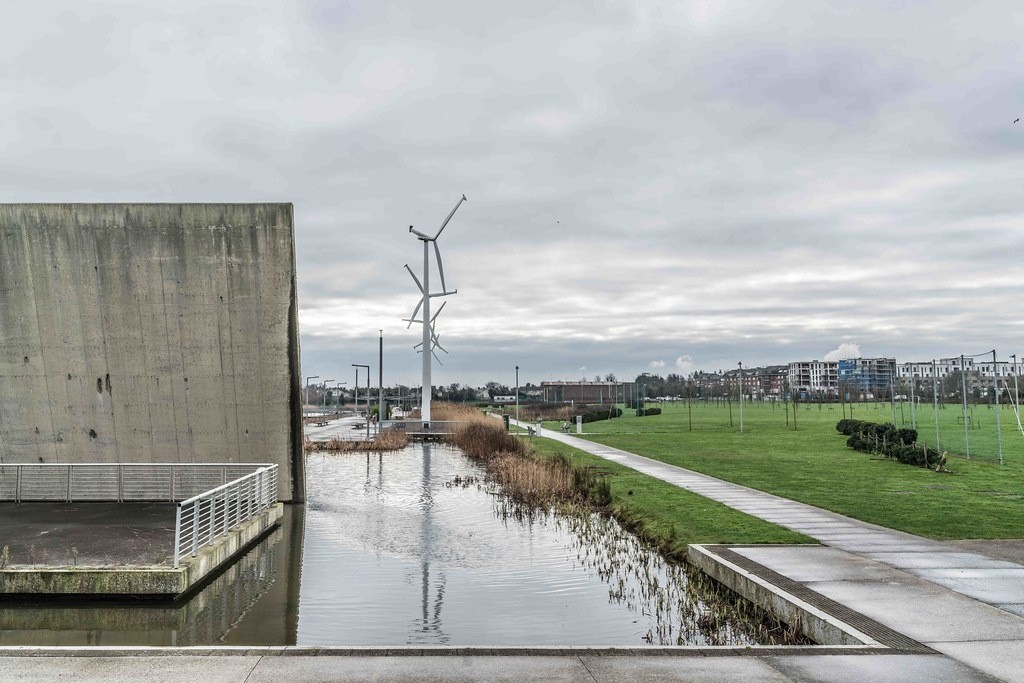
[324,380,335,424]
[352,364,370,437]
[307,376,319,425]
[337,383,347,420]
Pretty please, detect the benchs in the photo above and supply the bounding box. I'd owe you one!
[559,421,571,430]
[351,422,366,429]
[317,421,328,426]
[527,426,536,436]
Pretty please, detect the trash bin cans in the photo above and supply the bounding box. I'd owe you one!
[483,410,486,416]
[502,413,509,432]
